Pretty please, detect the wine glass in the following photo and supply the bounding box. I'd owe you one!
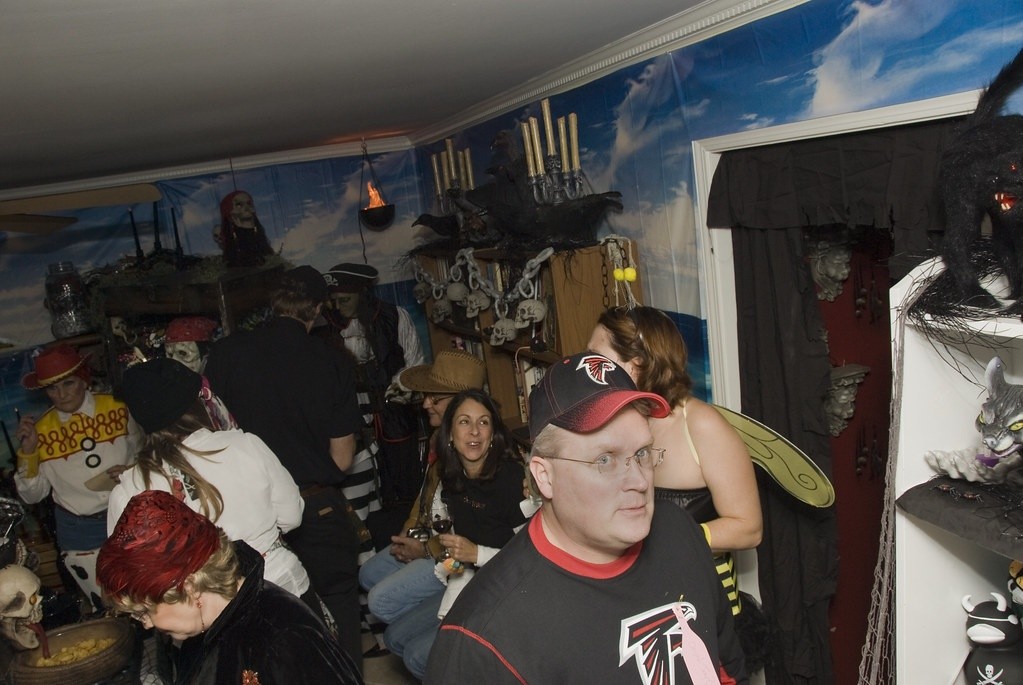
[426,498,458,561]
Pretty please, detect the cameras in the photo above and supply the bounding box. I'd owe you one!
[407,527,430,541]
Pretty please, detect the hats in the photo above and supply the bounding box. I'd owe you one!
[528,350,671,443]
[0,497,26,547]
[275,265,329,298]
[122,357,202,435]
[20,344,96,391]
[321,263,379,287]
[95,489,220,605]
[398,350,486,393]
[156,317,218,343]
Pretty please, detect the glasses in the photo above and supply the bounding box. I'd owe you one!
[419,391,454,405]
[407,519,455,543]
[540,448,666,477]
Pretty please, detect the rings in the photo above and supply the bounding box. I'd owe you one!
[445,549,450,557]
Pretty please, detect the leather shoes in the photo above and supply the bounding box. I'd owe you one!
[361,628,370,633]
[363,642,391,658]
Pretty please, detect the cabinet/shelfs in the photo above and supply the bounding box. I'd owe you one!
[887,257,1023,685]
[408,236,642,451]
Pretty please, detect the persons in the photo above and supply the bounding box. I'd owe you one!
[0,264,763,685]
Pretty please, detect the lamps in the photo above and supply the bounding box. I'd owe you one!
[520,98,583,206]
[358,143,396,232]
[431,137,478,216]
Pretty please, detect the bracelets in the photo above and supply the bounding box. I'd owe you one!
[424,542,432,557]
